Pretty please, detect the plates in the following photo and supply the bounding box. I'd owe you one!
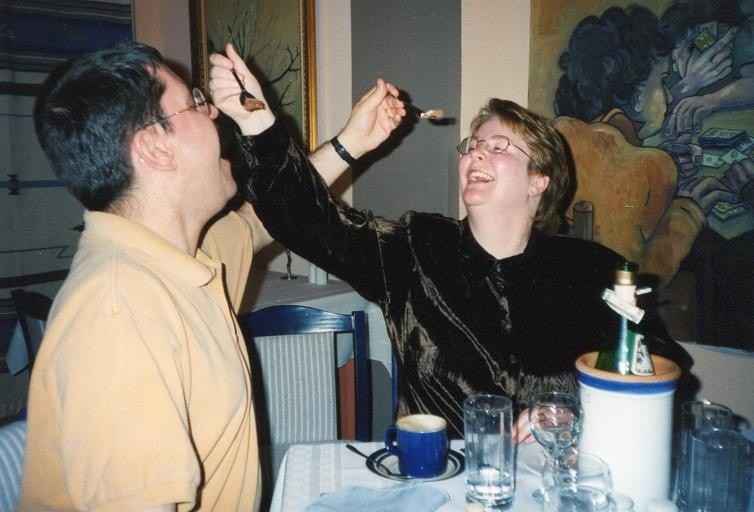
[364,444,466,483]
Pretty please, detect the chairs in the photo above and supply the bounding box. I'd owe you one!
[236,303,372,500]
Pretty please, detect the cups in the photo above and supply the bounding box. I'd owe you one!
[462,393,516,512]
[678,399,739,511]
[384,413,448,479]
[541,450,633,511]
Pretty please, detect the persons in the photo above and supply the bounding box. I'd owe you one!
[13,38,408,512]
[205,39,692,447]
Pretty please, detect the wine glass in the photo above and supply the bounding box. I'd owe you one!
[528,391,582,504]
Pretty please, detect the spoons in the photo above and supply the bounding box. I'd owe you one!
[218,48,266,116]
[344,441,411,478]
[377,86,456,128]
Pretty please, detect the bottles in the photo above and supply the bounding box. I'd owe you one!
[592,262,657,378]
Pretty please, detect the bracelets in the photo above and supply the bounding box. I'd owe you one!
[329,136,358,167]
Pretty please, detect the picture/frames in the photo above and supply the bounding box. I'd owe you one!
[184,0,317,165]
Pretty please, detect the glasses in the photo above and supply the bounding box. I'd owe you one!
[132,87,210,144]
[456,135,544,177]
